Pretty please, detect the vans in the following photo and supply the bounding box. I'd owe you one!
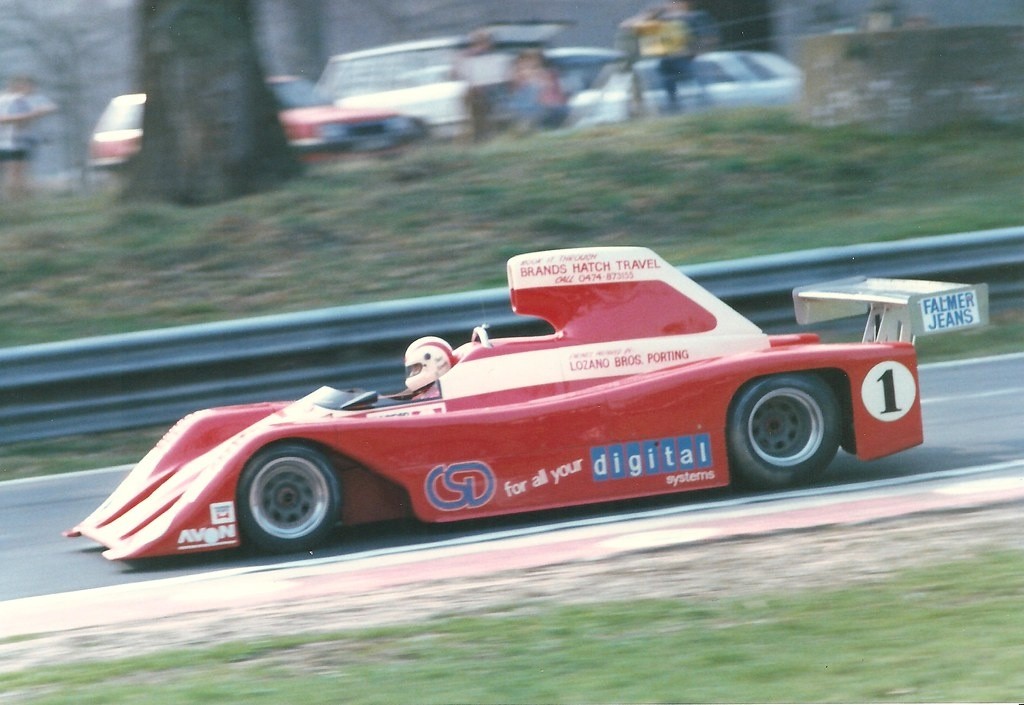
[317,33,581,144]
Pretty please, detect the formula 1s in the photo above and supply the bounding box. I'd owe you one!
[60,244,989,562]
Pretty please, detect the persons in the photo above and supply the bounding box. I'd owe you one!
[403,336,454,400]
[446,31,569,138]
[0,71,59,187]
[620,0,700,118]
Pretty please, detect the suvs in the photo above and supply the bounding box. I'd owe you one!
[83,75,429,174]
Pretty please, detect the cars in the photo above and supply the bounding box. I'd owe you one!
[541,51,805,125]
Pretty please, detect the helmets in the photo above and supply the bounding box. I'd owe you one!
[404,336,455,392]
